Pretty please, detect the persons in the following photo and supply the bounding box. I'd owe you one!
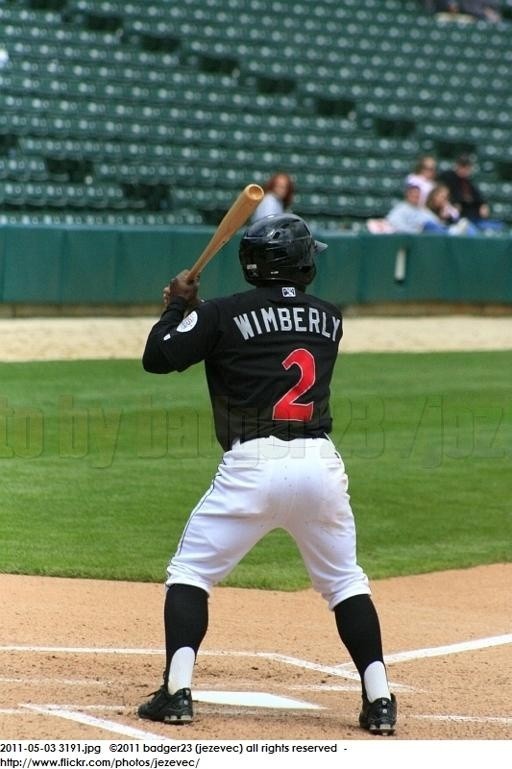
[385,155,503,237]
[422,0,503,26]
[248,174,294,222]
[136,213,398,735]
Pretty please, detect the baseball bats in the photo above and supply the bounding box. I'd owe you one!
[188,183,263,284]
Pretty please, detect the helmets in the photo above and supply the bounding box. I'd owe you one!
[239,212,328,287]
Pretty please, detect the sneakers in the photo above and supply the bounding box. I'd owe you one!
[360,694,398,735]
[139,685,193,723]
